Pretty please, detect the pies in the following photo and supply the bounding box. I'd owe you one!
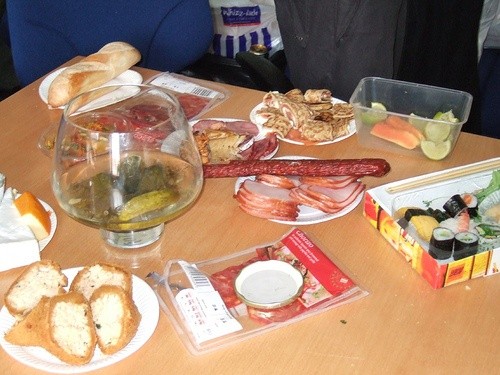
[254,88,354,143]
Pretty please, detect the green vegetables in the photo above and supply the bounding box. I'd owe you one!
[474,169,500,239]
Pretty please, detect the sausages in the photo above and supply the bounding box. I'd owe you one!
[201,158,391,178]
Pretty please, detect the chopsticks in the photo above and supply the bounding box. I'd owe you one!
[387,159,500,194]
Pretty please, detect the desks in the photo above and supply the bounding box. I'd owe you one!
[0,55,500,375]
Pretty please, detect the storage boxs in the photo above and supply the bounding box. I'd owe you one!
[362,156,500,290]
[346,76,473,162]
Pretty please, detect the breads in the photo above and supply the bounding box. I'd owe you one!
[179,127,243,165]
[47,41,141,107]
[14,191,51,240]
[3,259,141,366]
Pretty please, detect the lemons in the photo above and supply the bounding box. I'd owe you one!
[408,110,459,160]
[359,101,386,125]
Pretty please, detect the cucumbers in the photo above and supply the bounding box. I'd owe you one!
[62,154,182,222]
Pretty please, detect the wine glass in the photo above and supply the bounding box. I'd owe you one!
[50,81,204,289]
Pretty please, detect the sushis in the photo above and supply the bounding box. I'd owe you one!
[391,191,479,262]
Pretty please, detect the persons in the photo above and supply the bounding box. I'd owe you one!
[273,0,407,112]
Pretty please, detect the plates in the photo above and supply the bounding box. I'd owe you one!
[14,193,57,252]
[38,66,143,116]
[235,156,364,226]
[160,118,280,161]
[249,96,357,146]
[0,265,160,375]
[234,259,304,310]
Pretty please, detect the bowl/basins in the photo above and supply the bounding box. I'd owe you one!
[391,173,500,267]
[349,76,473,161]
